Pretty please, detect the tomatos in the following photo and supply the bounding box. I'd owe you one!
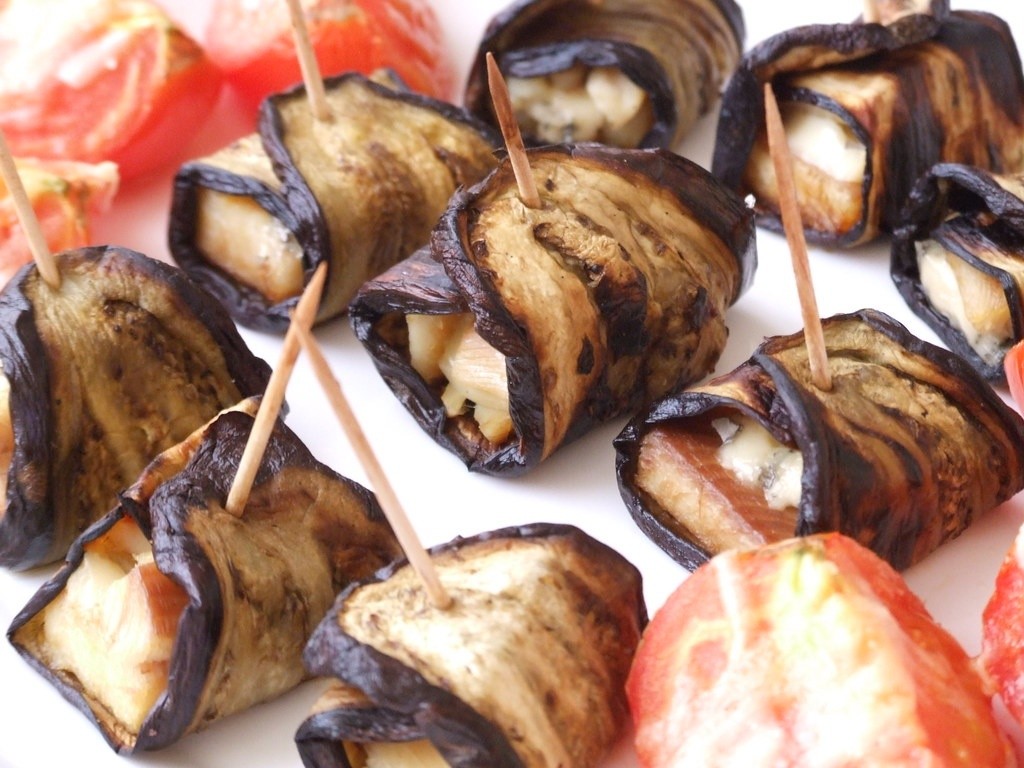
[0,0,450,265]
[618,521,1024,768]
